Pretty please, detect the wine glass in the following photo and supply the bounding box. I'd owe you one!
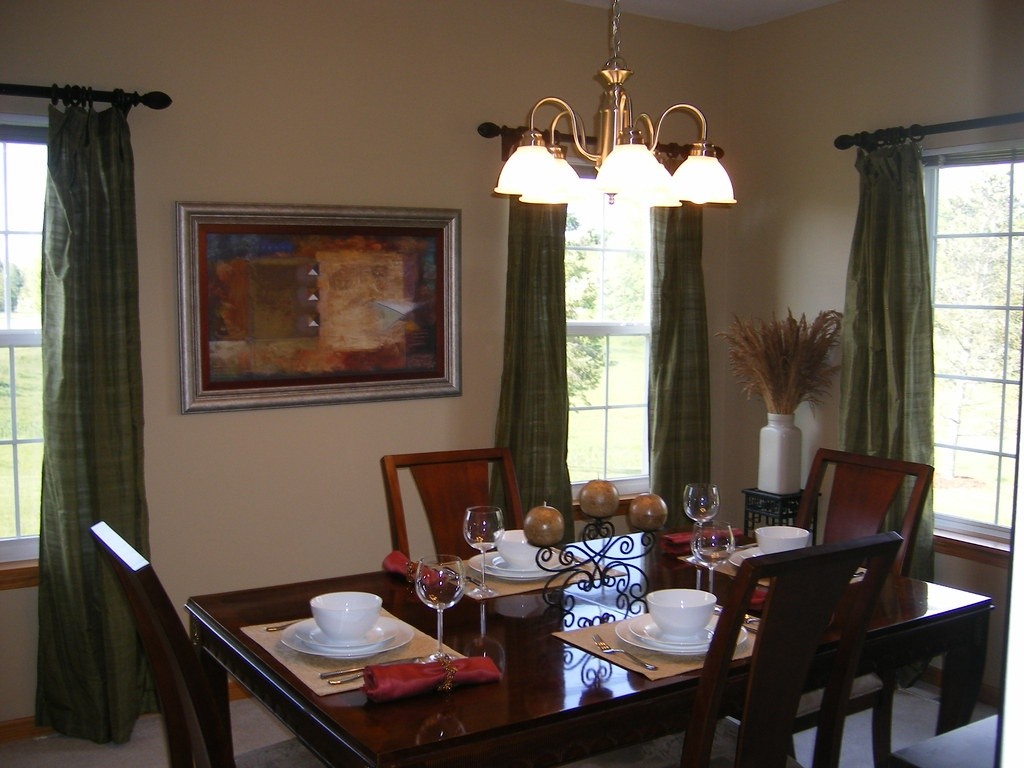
[691,521,735,612]
[683,483,719,564]
[413,554,465,664]
[462,505,504,599]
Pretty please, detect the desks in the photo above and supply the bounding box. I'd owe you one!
[182,533,995,768]
[741,489,822,546]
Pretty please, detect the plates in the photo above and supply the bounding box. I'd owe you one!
[615,614,748,655]
[295,620,398,647]
[628,614,720,646]
[468,552,574,580]
[728,552,743,566]
[740,546,763,559]
[484,552,561,573]
[281,618,414,658]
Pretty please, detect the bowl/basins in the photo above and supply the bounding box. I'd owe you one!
[309,591,383,638]
[755,526,810,554]
[497,529,546,568]
[647,589,717,635]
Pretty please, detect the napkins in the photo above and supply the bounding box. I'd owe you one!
[662,524,742,556]
[381,551,438,587]
[361,654,502,700]
[745,585,835,625]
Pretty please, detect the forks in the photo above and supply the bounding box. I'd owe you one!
[591,633,657,670]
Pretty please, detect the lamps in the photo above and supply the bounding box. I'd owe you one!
[493,0,740,208]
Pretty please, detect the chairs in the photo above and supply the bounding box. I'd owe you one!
[889,714,1000,767]
[93,521,334,768]
[381,447,524,578]
[565,532,904,768]
[726,449,934,768]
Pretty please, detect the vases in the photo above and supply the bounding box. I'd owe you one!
[758,409,803,495]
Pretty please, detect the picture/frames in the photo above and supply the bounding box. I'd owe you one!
[172,199,463,413]
